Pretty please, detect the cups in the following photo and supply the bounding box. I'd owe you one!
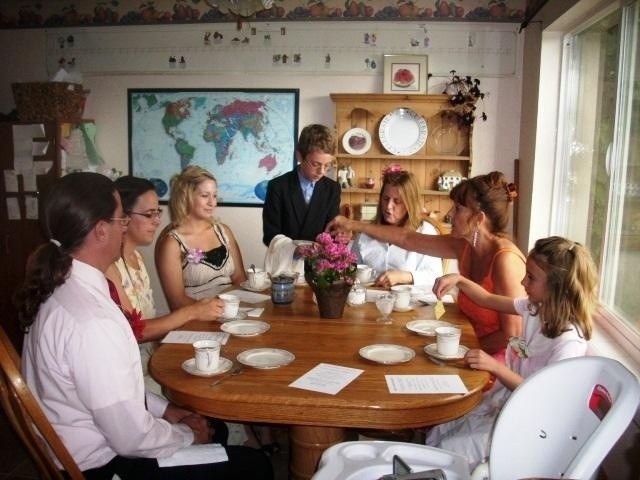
[215,293,240,319]
[391,286,411,308]
[436,328,463,357]
[269,275,295,304]
[364,177,375,188]
[357,264,376,281]
[193,339,221,372]
[247,268,266,288]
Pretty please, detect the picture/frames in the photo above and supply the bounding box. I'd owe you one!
[127,88,299,208]
[382,54,428,93]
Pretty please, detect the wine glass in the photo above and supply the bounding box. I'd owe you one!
[375,294,398,324]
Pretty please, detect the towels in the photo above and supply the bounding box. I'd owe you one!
[262,233,313,287]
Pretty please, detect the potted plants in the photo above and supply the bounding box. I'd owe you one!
[440,69,487,130]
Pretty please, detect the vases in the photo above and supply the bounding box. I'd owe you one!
[307,284,352,319]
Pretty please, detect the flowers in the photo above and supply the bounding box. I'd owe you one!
[293,229,357,285]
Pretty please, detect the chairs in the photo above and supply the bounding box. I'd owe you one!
[310,356,640,480]
[1,329,85,480]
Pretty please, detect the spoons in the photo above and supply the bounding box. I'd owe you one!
[209,365,244,387]
[427,356,476,372]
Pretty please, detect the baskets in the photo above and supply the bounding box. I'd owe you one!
[11,81,90,121]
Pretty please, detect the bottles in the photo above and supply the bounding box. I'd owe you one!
[345,204,355,220]
[345,280,368,306]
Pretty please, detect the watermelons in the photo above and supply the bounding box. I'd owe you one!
[393,69,415,88]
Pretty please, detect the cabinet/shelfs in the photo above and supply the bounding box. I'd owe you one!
[0,119,94,357]
[330,94,473,235]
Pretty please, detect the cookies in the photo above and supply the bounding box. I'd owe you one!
[434,299,445,320]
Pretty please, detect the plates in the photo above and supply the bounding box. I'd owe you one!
[378,107,428,156]
[405,318,457,336]
[342,128,372,154]
[358,342,413,367]
[218,319,271,338]
[424,341,470,360]
[430,122,467,156]
[180,357,234,378]
[240,279,272,292]
[238,347,296,371]
[392,306,416,313]
[419,295,454,307]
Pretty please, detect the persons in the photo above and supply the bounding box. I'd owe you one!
[424,237,601,479]
[104,175,249,448]
[323,171,529,393]
[342,171,445,288]
[261,123,343,259]
[12,172,275,479]
[153,164,282,457]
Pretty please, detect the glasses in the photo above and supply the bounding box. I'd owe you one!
[130,209,161,218]
[110,214,131,225]
